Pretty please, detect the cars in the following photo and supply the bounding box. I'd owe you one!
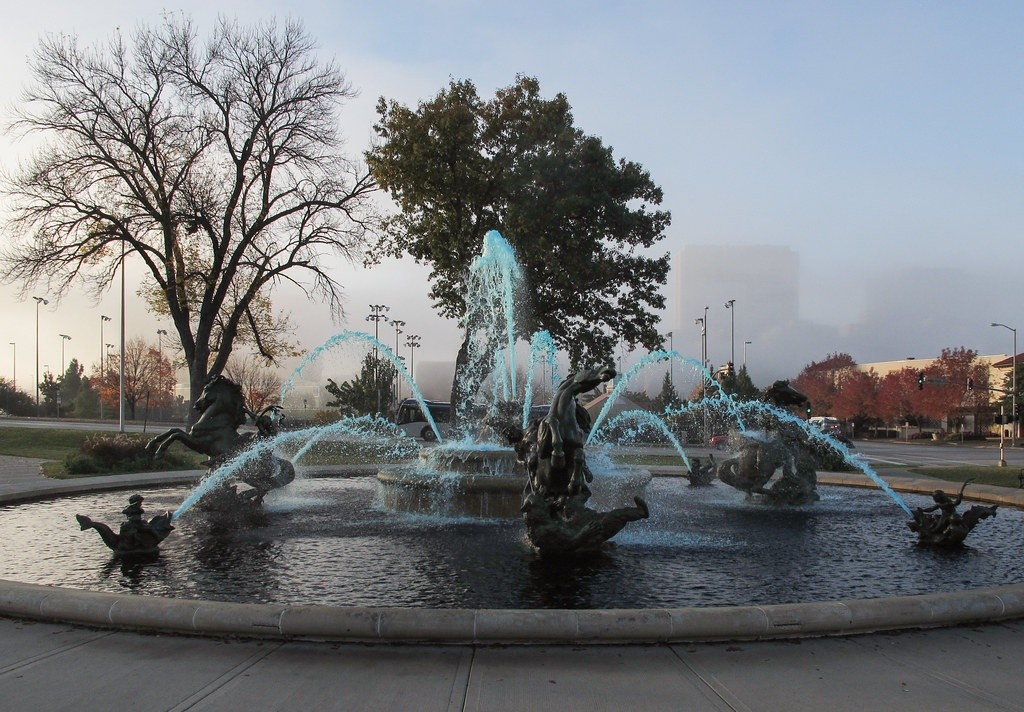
[709,435,727,450]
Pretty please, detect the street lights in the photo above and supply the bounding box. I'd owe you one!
[107,218,134,435]
[989,321,1017,446]
[99,314,111,419]
[665,331,673,385]
[743,341,751,365]
[32,296,49,419]
[388,319,405,412]
[722,299,737,364]
[402,334,421,398]
[363,304,390,412]
[693,306,709,445]
[59,333,72,378]
[9,342,16,391]
[105,342,114,371]
[155,328,167,401]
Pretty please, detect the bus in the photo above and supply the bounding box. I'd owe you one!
[395,396,451,442]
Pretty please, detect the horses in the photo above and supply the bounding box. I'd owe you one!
[144,375,296,507]
[523,367,617,500]
[718,379,808,499]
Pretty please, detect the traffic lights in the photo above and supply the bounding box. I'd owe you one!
[806,401,811,419]
[917,371,923,391]
[967,378,974,391]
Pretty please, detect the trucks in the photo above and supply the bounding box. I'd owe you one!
[808,417,842,435]
